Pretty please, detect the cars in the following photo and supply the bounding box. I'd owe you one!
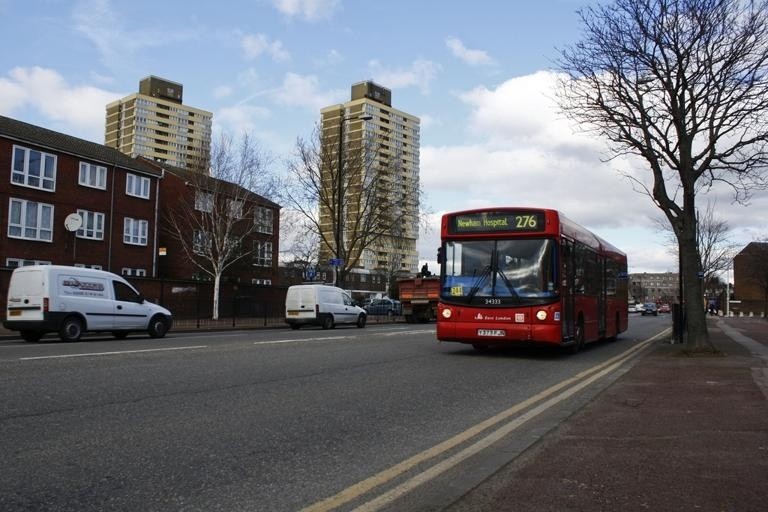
[362,297,401,317]
[628,300,670,316]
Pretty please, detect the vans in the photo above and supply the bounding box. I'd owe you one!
[2,263,173,343]
[285,284,368,330]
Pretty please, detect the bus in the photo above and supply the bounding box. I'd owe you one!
[436,206,630,355]
